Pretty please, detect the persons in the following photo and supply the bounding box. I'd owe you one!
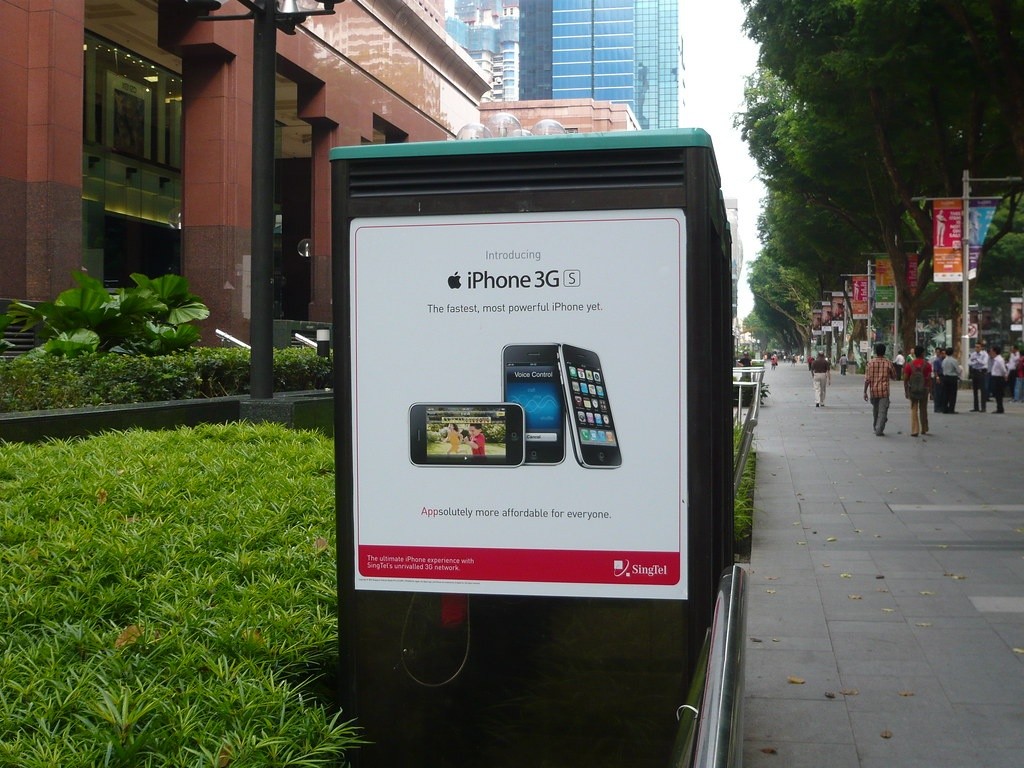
[863,343,897,436]
[936,209,947,247]
[892,341,1024,413]
[903,345,932,437]
[770,353,813,371]
[440,423,485,456]
[854,281,859,297]
[738,352,751,378]
[810,350,830,407]
[839,353,848,375]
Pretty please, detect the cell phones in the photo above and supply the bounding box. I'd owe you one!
[556,342,623,469]
[408,402,526,468]
[501,342,567,466]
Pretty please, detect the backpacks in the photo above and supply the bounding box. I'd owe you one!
[905,360,928,401]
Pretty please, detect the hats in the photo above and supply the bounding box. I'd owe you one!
[744,352,747,354]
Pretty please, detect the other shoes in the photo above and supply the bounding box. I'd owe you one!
[821,404,824,406]
[969,409,979,412]
[873,431,876,433]
[921,432,926,434]
[986,398,993,402]
[991,410,1004,414]
[816,402,820,407]
[978,410,986,413]
[1009,398,1018,402]
[910,433,918,437]
[876,433,885,436]
[948,411,959,414]
